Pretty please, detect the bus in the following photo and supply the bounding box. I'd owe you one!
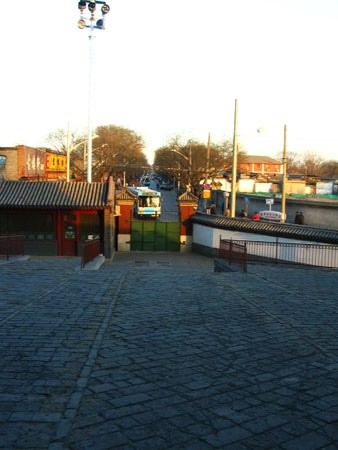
[128,186,164,218]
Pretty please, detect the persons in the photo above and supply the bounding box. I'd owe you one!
[294,211,304,225]
[222,201,230,214]
[250,212,260,220]
[238,210,248,218]
[226,208,230,217]
[210,201,217,214]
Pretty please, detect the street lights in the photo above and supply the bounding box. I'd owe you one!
[76,0,112,183]
[170,149,192,188]
[67,134,100,182]
[84,143,108,179]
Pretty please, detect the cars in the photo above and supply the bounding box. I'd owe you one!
[139,172,171,190]
